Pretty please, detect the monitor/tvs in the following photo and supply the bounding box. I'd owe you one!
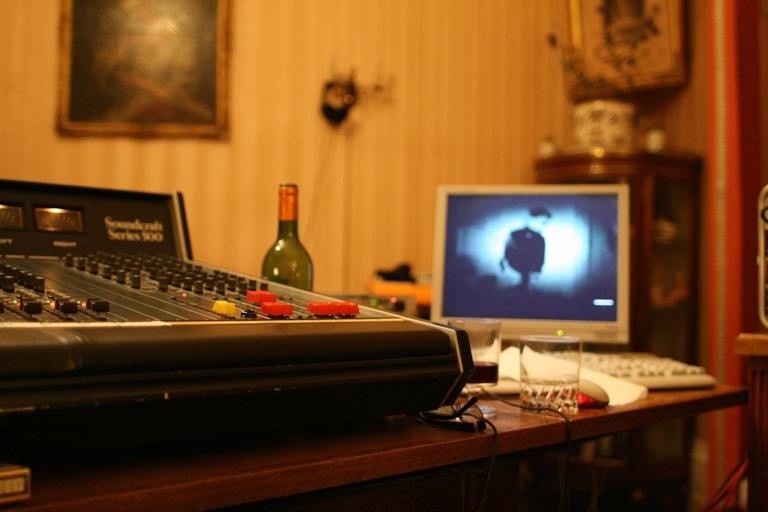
[431,184,631,347]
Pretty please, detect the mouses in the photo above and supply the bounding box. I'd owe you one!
[561,382,608,406]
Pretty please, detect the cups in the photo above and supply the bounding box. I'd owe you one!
[519,336,583,419]
[446,316,502,389]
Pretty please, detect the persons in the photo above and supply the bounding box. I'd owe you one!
[495,204,553,294]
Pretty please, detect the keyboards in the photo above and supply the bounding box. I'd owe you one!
[463,350,719,398]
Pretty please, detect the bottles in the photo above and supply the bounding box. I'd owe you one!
[261,183,314,293]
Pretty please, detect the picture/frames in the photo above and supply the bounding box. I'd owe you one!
[58,1,231,136]
